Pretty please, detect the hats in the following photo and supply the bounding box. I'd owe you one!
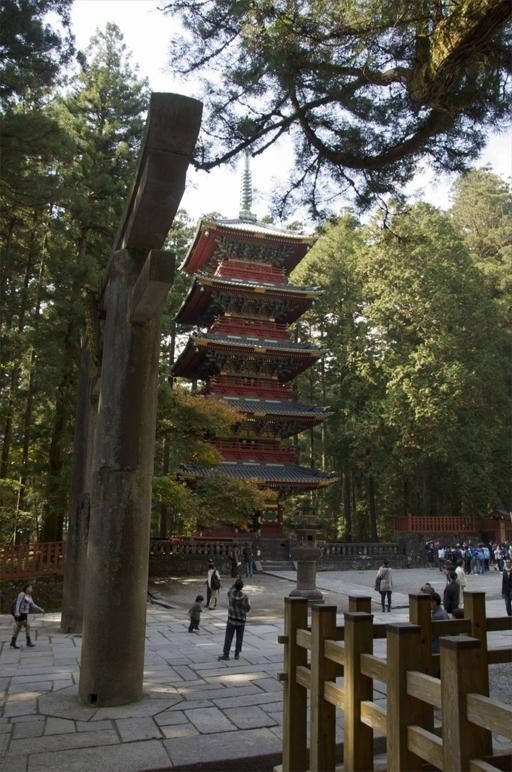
[430,591,441,602]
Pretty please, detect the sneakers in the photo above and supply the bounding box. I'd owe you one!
[219,654,239,660]
[189,627,199,632]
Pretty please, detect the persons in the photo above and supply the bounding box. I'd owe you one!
[223,544,241,579]
[216,578,252,661]
[9,582,45,650]
[375,558,394,614]
[184,594,204,634]
[203,558,222,611]
[242,548,255,577]
[422,590,450,682]
[417,537,512,619]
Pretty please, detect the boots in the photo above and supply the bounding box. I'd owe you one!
[10,637,20,649]
[26,636,36,647]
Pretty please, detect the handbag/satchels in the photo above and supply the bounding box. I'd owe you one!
[376,578,381,590]
[11,599,16,616]
[211,573,220,590]
